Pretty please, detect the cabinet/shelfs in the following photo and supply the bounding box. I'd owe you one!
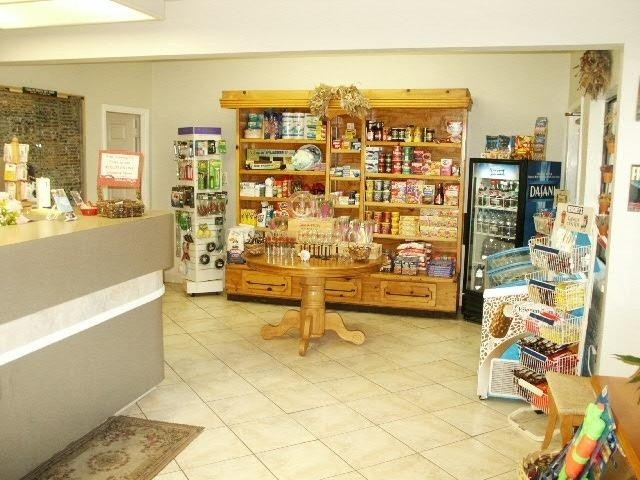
[221,89,473,315]
[507,206,598,442]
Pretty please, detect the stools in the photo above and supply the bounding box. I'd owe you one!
[541,368,597,450]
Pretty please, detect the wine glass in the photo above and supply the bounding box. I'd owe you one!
[298,229,342,260]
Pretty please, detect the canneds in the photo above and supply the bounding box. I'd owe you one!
[392,127,404,141]
[423,127,435,142]
[378,152,392,173]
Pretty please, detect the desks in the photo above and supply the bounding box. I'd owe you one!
[237,250,383,356]
[590,373,640,480]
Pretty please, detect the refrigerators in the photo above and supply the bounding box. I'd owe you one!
[476,246,606,402]
[462,157,562,325]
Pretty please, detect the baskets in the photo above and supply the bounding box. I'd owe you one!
[528,214,593,276]
[512,270,587,414]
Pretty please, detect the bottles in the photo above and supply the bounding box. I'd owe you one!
[474,179,518,289]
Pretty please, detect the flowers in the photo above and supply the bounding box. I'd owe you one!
[572,50,613,103]
